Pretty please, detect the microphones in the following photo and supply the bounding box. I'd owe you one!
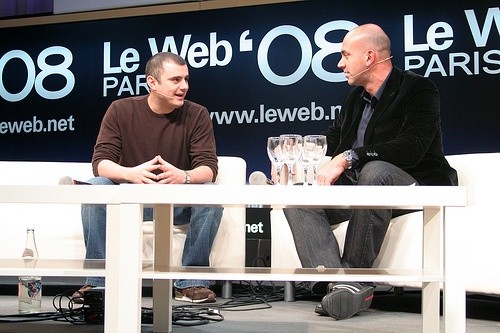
[249,171,276,186]
[155,90,174,98]
[350,56,394,79]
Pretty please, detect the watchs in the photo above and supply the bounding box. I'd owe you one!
[181,169,192,185]
[344,150,354,170]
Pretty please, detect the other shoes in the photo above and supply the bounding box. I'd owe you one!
[315,302,329,316]
[321,280,374,320]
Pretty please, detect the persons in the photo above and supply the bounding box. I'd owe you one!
[71,53,224,303]
[283,23,459,320]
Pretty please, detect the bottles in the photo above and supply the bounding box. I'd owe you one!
[18,226,42,314]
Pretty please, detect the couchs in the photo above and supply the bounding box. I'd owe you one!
[270,151,500,304]
[0,152,247,298]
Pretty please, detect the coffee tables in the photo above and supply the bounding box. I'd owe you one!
[0,182,472,333]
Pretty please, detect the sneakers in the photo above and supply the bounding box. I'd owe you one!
[176,286,217,304]
[71,285,93,304]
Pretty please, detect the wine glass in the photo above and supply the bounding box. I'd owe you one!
[267,137,291,185]
[299,137,317,186]
[303,135,327,186]
[279,134,303,186]
